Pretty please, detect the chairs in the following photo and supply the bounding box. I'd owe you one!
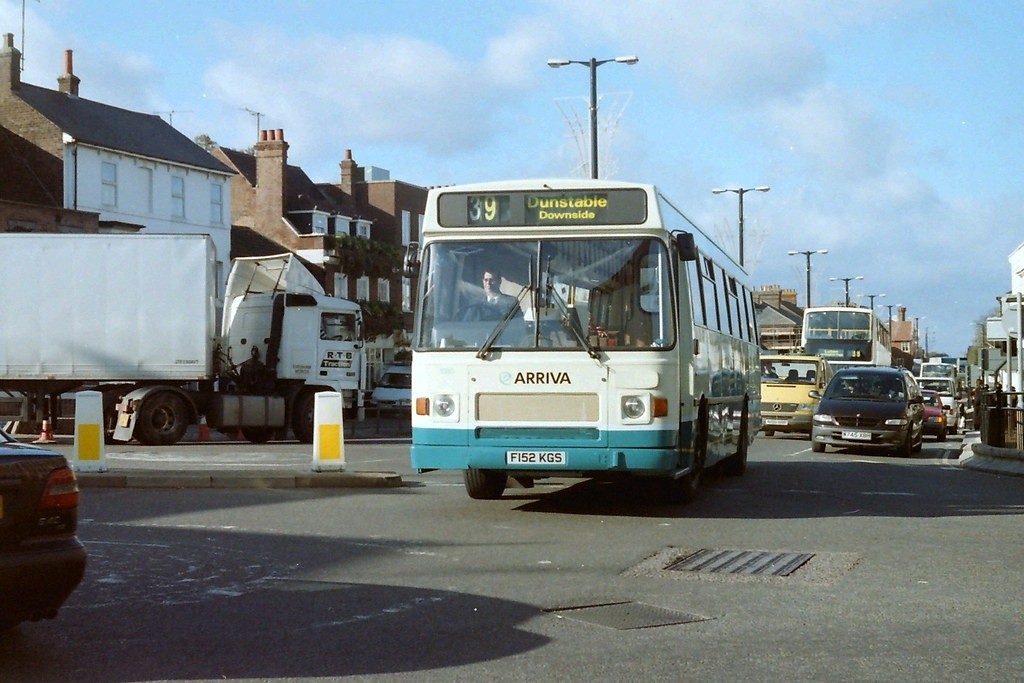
[523,306,574,350]
[764,367,818,387]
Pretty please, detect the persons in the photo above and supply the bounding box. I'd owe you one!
[956,379,1018,427]
[762,362,778,380]
[462,264,526,348]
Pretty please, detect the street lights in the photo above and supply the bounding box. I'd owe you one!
[788,250,926,360]
[710,187,770,267]
[546,55,639,178]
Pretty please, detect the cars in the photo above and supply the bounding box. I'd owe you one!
[759,356,960,457]
[0,429,87,630]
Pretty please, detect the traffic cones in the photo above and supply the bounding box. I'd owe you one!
[198,416,212,442]
[31,418,57,444]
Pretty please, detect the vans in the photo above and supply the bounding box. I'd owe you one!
[370,365,411,417]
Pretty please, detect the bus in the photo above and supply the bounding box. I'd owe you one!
[410,178,760,500]
[799,305,893,375]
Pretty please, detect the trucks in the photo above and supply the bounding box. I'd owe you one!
[1,231,365,447]
